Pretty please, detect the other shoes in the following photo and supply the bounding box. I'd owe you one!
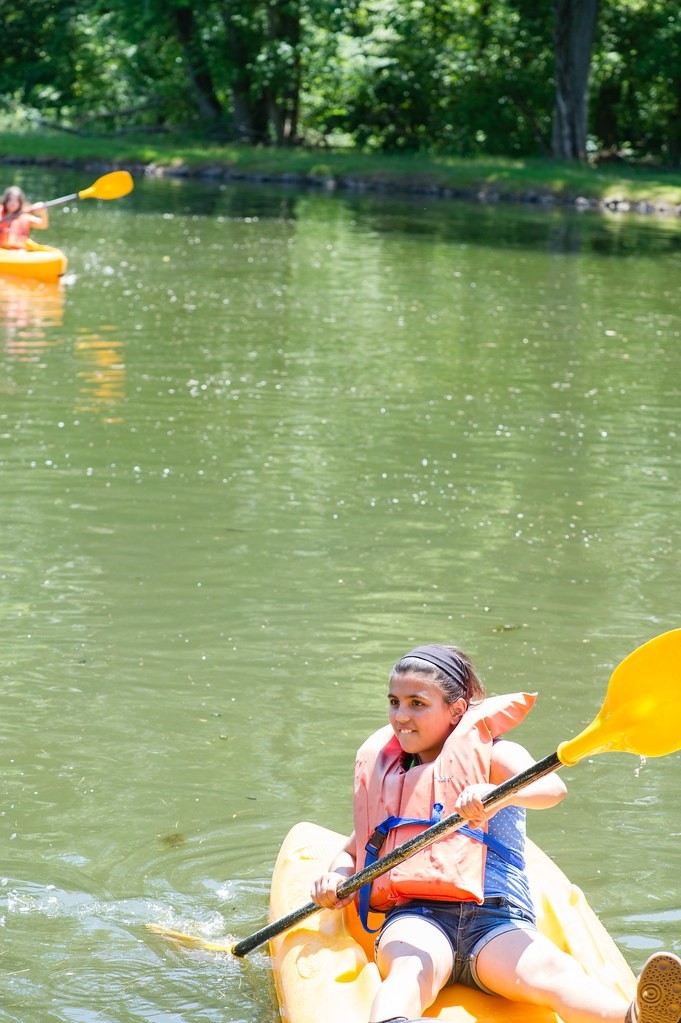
[630,950,681,1023]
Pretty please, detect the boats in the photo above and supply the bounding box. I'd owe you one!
[268,822,638,1023]
[0,237,66,282]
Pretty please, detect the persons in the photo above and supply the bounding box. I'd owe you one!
[0,186,48,250]
[311,645,681,1023]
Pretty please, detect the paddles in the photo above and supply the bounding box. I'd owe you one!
[146,626,681,961]
[0,170,134,223]
